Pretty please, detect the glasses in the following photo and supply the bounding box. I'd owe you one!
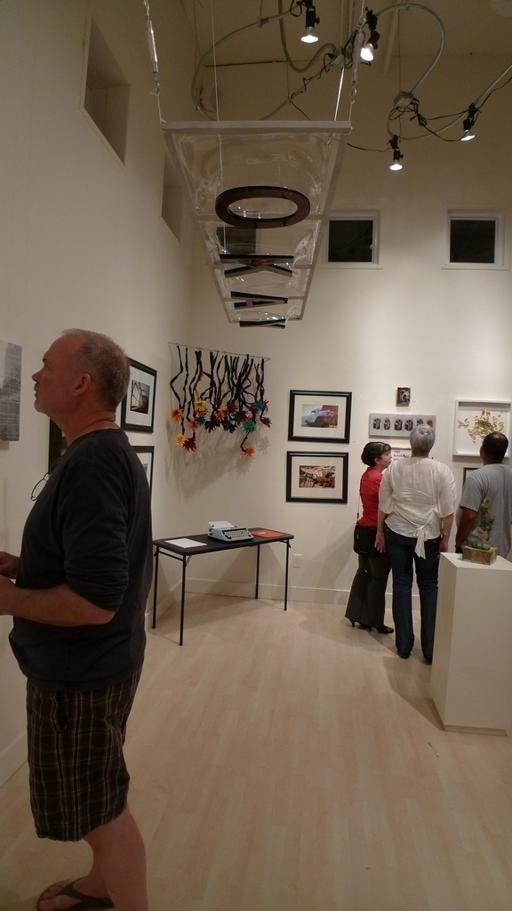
[29,459,67,502]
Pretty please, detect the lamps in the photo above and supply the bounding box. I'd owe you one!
[355,9,382,62]
[295,1,324,43]
[460,104,483,141]
[386,136,406,172]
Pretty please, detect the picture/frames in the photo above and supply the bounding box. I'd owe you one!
[284,389,353,444]
[368,409,436,441]
[284,453,351,504]
[398,388,410,406]
[453,398,509,461]
[373,446,416,473]
[124,444,157,501]
[119,361,158,432]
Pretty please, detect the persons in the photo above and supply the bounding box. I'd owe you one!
[457,432,512,560]
[0,327,150,907]
[345,440,393,636]
[312,467,324,487]
[375,424,455,665]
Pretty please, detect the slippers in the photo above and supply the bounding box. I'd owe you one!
[34,874,115,910]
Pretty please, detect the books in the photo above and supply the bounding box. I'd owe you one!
[251,529,285,540]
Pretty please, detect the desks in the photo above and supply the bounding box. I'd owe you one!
[147,526,295,647]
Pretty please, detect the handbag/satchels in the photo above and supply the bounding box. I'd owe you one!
[353,476,388,558]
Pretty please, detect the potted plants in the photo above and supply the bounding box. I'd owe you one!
[457,489,506,566]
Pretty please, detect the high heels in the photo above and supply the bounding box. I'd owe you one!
[364,622,396,634]
[344,614,364,628]
